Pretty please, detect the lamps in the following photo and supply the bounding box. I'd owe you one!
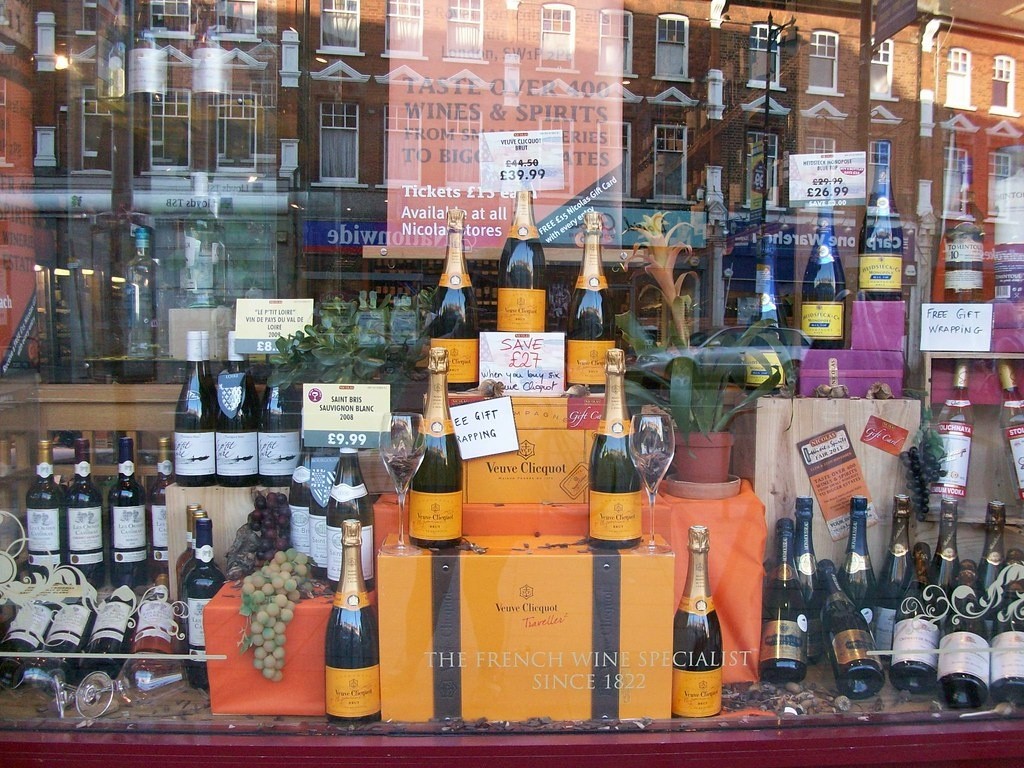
[129,48,166,103]
[192,46,232,98]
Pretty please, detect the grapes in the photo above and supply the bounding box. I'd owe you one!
[249,491,293,560]
[900,441,947,522]
[241,547,313,683]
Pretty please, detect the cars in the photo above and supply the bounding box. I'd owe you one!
[633,325,814,391]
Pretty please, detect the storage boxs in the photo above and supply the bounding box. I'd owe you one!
[146,294,1023,738]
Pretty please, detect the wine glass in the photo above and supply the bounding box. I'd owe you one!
[627,414,674,555]
[75,653,188,717]
[379,412,426,556]
[0,650,118,719]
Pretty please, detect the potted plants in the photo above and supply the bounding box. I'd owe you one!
[617,300,810,499]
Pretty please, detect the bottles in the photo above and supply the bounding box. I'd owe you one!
[927,360,973,512]
[937,559,990,709]
[990,548,1024,698]
[795,498,826,665]
[588,348,642,550]
[879,494,911,661]
[996,359,1024,518]
[977,500,1007,630]
[931,499,959,614]
[740,141,986,395]
[671,525,723,718]
[839,497,879,640]
[432,190,614,391]
[409,347,464,547]
[27,174,372,594]
[816,559,885,700]
[757,517,807,682]
[0,573,169,690]
[176,505,378,721]
[888,542,941,694]
